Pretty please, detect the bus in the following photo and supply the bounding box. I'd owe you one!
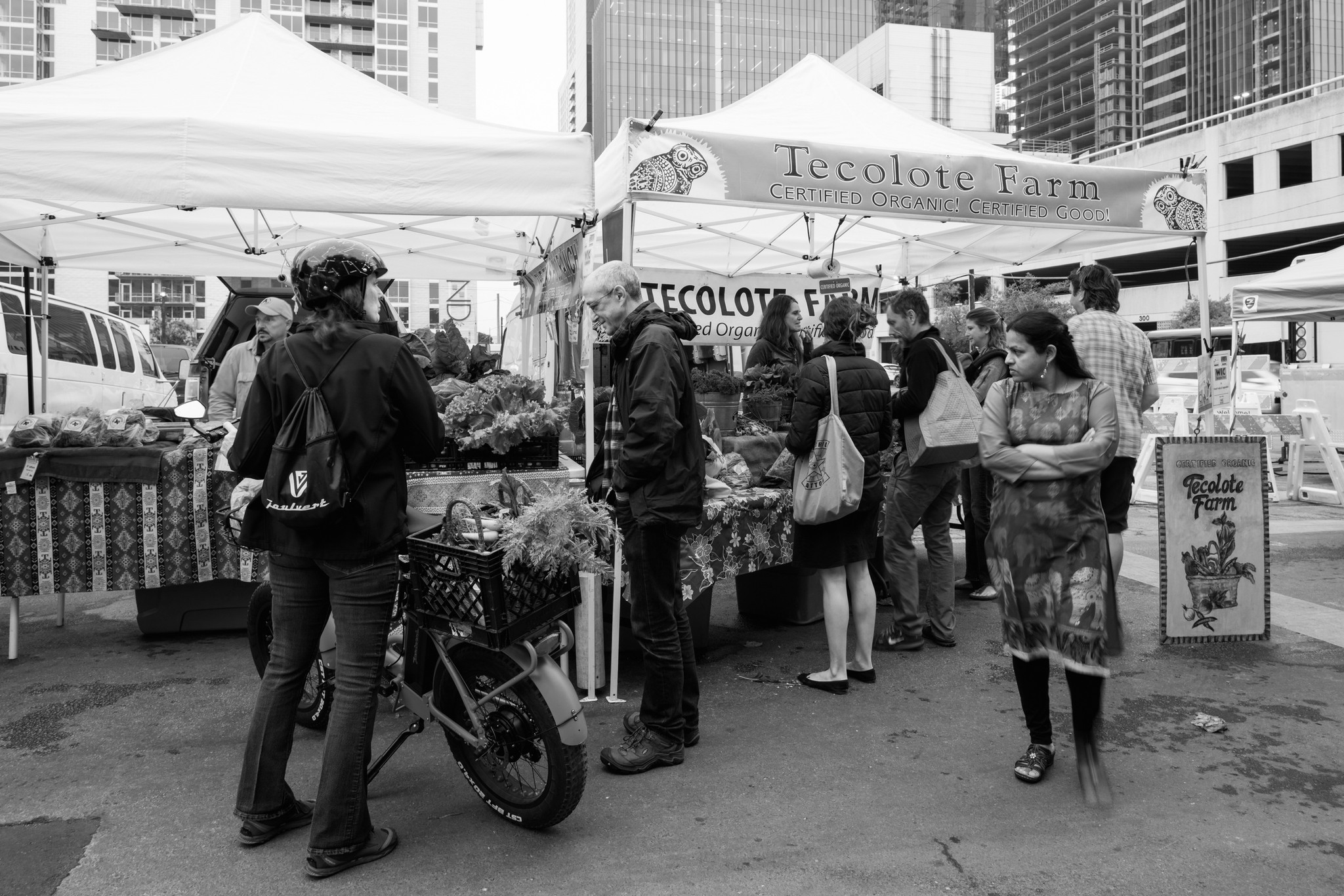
[1146,326,1283,378]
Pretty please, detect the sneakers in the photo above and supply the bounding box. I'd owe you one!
[872,623,925,651]
[600,727,685,771]
[623,711,701,748]
[238,799,319,843]
[303,826,398,877]
[923,618,957,647]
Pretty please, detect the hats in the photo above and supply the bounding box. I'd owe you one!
[245,296,294,322]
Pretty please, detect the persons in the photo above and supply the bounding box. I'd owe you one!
[786,292,898,697]
[1060,259,1162,587]
[956,308,1011,599]
[976,312,1124,794]
[573,258,714,772]
[880,292,967,651]
[225,235,448,872]
[207,295,293,435]
[739,295,815,428]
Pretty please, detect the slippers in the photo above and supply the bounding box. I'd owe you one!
[1013,744,1056,783]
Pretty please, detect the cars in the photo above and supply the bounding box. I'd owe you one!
[880,363,902,388]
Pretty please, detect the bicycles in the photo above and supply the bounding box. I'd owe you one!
[177,400,594,833]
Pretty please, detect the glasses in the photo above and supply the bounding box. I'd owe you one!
[587,289,614,311]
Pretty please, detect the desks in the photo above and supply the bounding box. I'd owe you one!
[679,431,824,660]
[1,428,583,660]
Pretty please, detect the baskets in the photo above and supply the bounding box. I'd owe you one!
[216,506,266,552]
[405,502,582,652]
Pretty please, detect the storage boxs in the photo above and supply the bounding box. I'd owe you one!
[403,435,560,472]
[406,475,583,652]
[695,390,783,435]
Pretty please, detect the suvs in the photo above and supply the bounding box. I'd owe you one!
[183,274,407,417]
[150,344,193,400]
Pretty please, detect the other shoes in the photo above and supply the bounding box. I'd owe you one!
[954,578,999,600]
[797,673,849,695]
[846,668,877,683]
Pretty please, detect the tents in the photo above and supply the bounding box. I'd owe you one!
[573,45,1212,398]
[0,13,602,475]
[1229,246,1344,402]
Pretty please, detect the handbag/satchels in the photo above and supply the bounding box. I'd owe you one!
[793,354,865,525]
[896,336,984,467]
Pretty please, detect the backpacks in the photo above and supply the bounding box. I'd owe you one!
[259,331,378,524]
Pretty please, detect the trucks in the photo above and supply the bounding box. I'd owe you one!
[494,278,744,458]
[0,280,180,445]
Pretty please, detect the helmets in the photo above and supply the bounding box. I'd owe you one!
[289,238,388,311]
